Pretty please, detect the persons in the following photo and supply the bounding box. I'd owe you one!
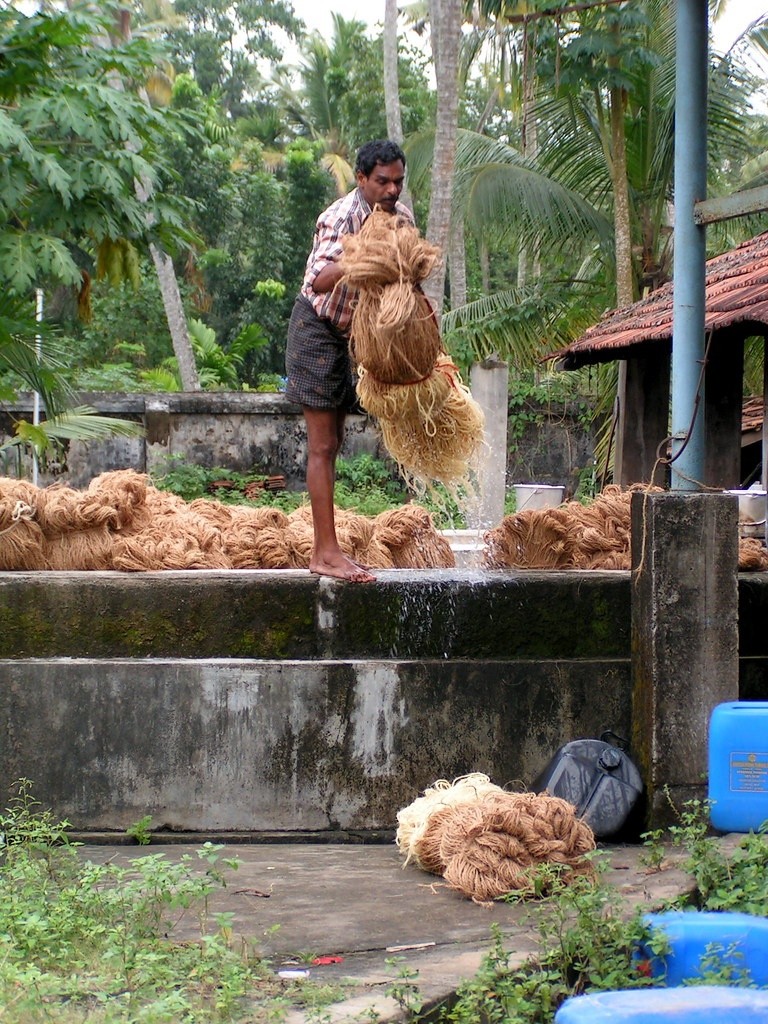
[285,139,418,582]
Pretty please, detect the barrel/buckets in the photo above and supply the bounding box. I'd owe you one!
[512,483,565,516]
[723,489,767,537]
